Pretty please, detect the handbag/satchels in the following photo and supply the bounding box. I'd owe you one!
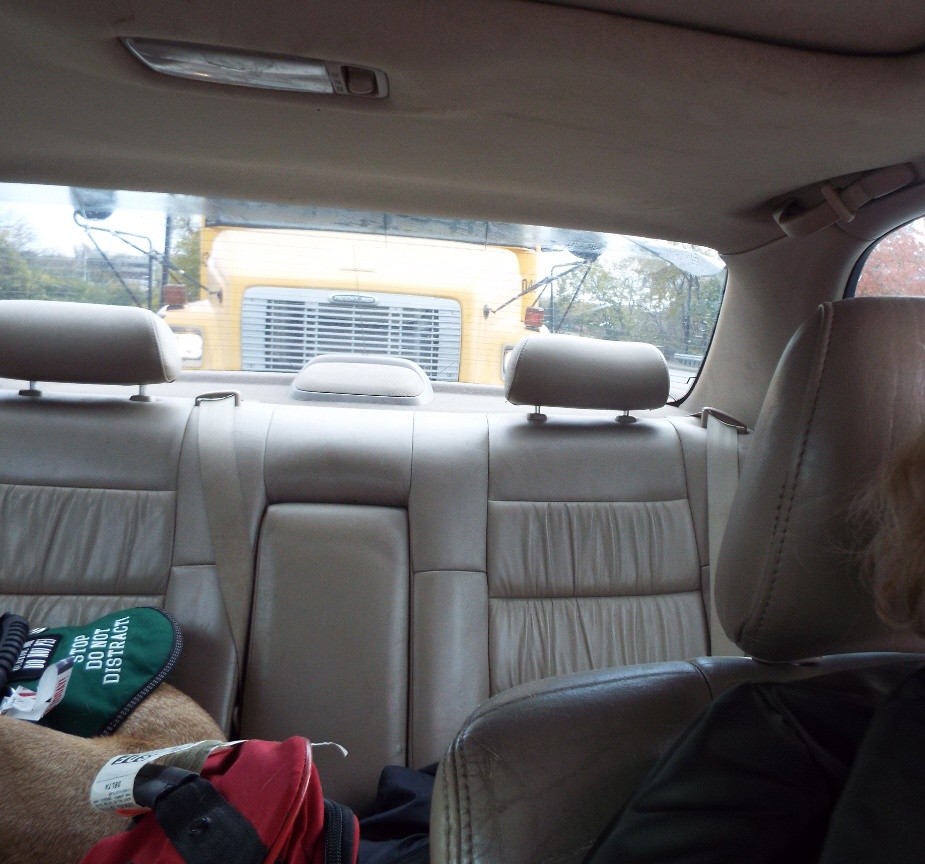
[77,735,361,864]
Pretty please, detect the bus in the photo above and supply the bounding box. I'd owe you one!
[68,185,601,391]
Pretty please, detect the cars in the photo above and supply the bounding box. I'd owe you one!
[0,0,925,864]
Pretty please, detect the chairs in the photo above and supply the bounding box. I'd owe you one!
[416,290,925,864]
[2,294,235,727]
[439,331,729,701]
